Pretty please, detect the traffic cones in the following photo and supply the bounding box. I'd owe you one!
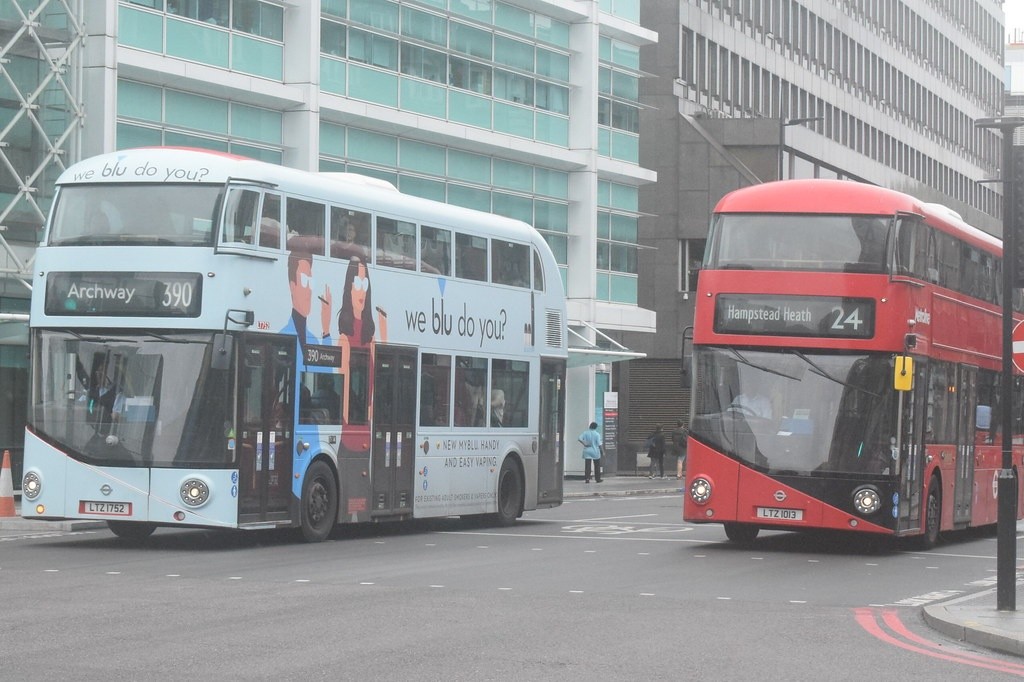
[0,450,21,516]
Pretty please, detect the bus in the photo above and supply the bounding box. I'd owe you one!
[676,180,1024,540]
[13,144,569,537]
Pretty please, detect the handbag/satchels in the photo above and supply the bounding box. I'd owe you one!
[680,429,687,448]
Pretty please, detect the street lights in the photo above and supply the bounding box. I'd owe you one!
[972,117,1024,605]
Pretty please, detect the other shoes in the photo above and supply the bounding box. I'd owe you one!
[677,475,682,480]
[586,479,589,483]
[649,475,655,479]
[596,479,603,483]
[661,475,667,479]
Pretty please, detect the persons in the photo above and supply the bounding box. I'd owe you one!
[578,422,605,483]
[299,374,342,423]
[727,377,773,419]
[434,374,505,426]
[649,424,668,480]
[345,223,358,243]
[79,364,128,420]
[672,419,688,480]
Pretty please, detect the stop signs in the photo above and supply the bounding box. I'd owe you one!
[1010,321,1024,373]
